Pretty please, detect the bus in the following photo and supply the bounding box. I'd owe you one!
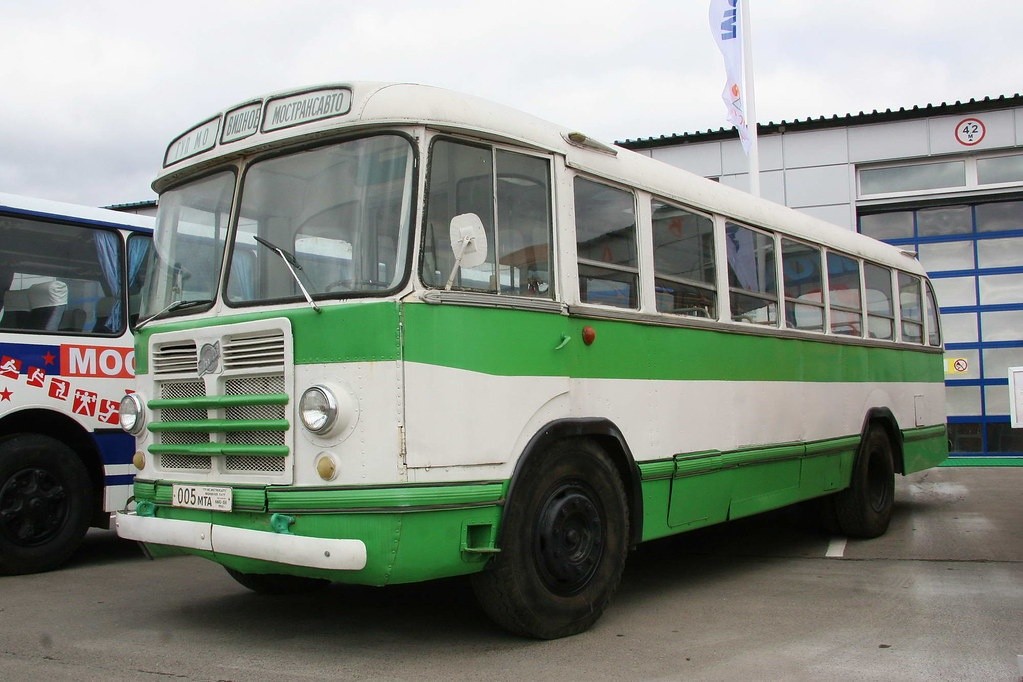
[0,189,527,574]
[110,74,950,639]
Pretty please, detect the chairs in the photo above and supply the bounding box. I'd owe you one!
[668,307,858,337]
[1,280,118,332]
